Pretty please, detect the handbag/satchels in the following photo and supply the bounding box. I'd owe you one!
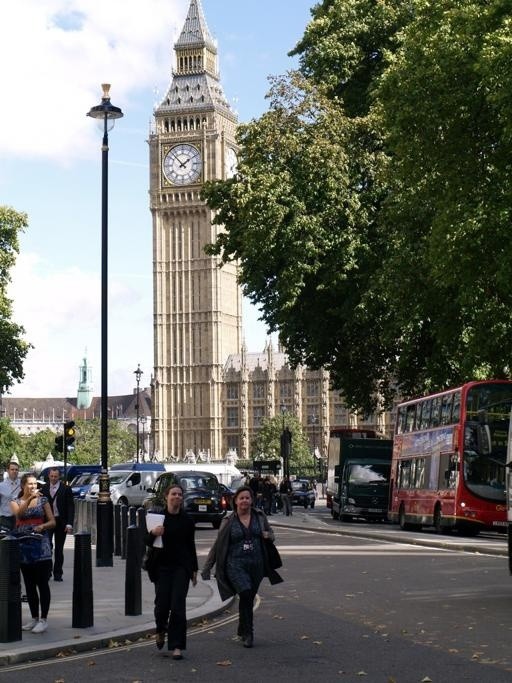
[260,534,280,561]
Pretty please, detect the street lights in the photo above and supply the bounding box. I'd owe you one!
[87,82,124,566]
[311,412,317,481]
[280,399,287,477]
[133,363,143,464]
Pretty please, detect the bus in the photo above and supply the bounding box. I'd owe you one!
[387,379,511,537]
[328,428,390,509]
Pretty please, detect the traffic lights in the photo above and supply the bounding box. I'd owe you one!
[64,421,75,453]
[53,435,62,452]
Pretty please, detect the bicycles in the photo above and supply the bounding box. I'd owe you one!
[0,526,45,601]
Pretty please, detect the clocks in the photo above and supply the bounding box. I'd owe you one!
[161,142,203,187]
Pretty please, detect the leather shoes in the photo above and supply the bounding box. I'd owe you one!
[172,652,182,660]
[244,633,253,648]
[155,634,165,649]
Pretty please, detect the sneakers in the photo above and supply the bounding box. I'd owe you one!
[32,619,49,633]
[21,618,38,631]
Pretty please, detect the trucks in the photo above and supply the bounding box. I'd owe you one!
[326,437,393,523]
[37,463,318,510]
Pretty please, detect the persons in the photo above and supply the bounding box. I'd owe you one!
[249,472,292,516]
[40,468,75,581]
[142,484,198,659]
[201,487,283,648]
[0,462,22,531]
[10,474,56,633]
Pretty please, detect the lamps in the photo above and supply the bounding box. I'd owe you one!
[224,146,240,182]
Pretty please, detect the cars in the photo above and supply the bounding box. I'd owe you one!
[140,470,227,529]
